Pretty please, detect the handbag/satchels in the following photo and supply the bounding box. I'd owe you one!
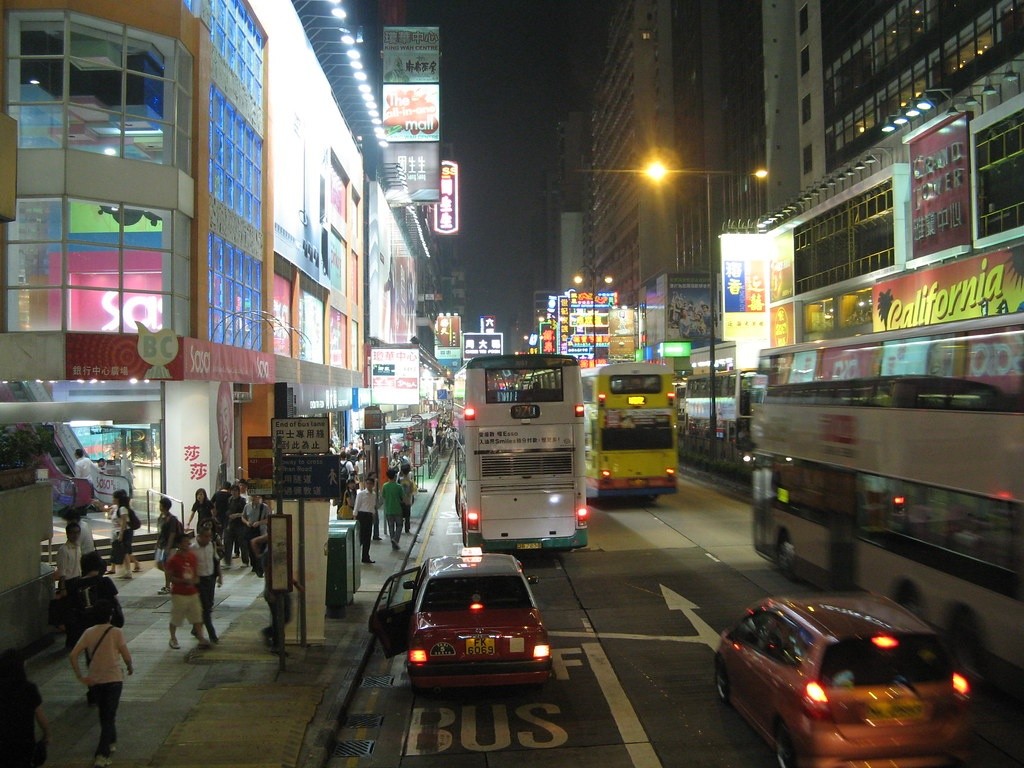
[338,504,354,518]
[243,525,260,541]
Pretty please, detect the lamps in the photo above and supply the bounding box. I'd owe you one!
[964,83,1003,106]
[29,71,40,85]
[332,84,371,93]
[350,125,385,134]
[349,117,382,125]
[318,49,361,60]
[980,72,1021,95]
[323,61,364,70]
[293,0,355,48]
[355,133,387,139]
[360,141,389,150]
[343,109,380,118]
[334,93,375,101]
[882,88,954,133]
[756,146,894,232]
[1003,57,1024,81]
[327,72,367,81]
[377,163,409,197]
[339,101,377,109]
[946,94,983,116]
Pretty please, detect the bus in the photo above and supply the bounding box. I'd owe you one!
[675,383,688,421]
[747,308,1024,711]
[523,362,680,507]
[682,366,761,462]
[451,353,589,559]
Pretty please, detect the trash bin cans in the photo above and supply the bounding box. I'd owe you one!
[325,521,361,618]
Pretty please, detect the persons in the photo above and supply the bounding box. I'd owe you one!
[533,382,541,389]
[98,458,106,471]
[156,499,179,594]
[167,535,210,648]
[70,600,134,768]
[74,449,102,500]
[65,551,125,706]
[0,648,52,768]
[325,440,416,563]
[426,418,458,454]
[188,479,306,657]
[57,514,93,631]
[190,527,222,644]
[89,489,142,578]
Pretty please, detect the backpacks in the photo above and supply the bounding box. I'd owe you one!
[166,515,184,543]
[117,507,141,530]
[340,461,348,480]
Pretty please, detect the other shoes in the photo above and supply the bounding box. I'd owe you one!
[210,633,219,643]
[232,553,240,559]
[361,558,376,563]
[406,529,409,533]
[108,742,116,751]
[373,537,382,541]
[223,565,232,570]
[157,587,172,594]
[390,539,400,551]
[195,632,209,646]
[169,637,180,648]
[240,563,249,567]
[261,628,289,657]
[105,569,116,574]
[131,567,142,572]
[96,756,112,767]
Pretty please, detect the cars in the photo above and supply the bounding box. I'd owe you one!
[712,591,980,768]
[368,547,552,702]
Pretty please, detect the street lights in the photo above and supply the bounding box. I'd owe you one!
[649,163,772,460]
[572,263,613,365]
